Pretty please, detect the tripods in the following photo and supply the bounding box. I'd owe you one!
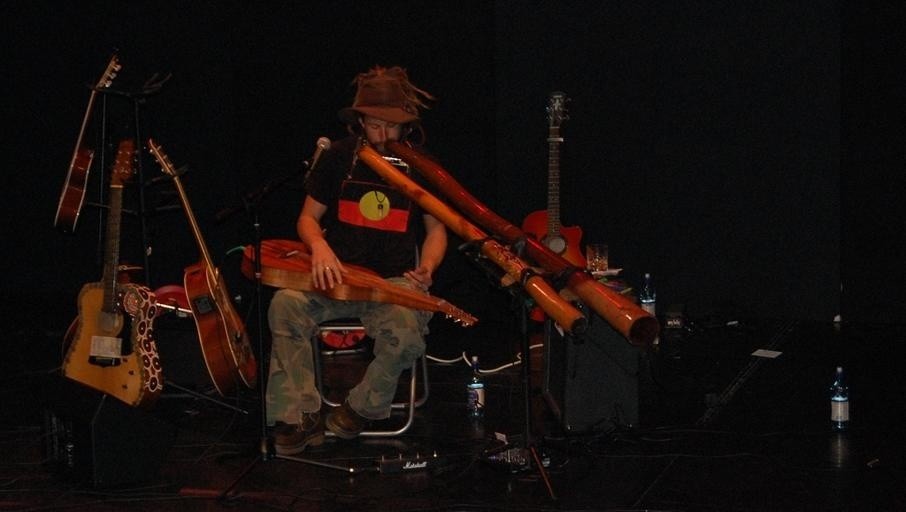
[215,162,361,503]
[459,243,595,502]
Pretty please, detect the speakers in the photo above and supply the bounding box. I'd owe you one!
[43,377,161,489]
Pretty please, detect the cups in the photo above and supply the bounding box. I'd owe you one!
[585,244,609,273]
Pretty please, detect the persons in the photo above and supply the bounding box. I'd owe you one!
[265,67,448,454]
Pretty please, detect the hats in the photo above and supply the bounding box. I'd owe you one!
[338,67,418,123]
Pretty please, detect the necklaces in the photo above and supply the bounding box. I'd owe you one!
[375,189,387,217]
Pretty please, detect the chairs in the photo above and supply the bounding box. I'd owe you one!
[301,238,431,440]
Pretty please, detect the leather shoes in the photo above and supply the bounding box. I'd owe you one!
[261,411,323,457]
[324,400,368,439]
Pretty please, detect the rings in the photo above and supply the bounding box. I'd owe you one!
[324,267,330,270]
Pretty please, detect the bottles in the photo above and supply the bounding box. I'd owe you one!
[463,356,487,419]
[830,366,850,433]
[640,272,656,316]
[486,448,550,468]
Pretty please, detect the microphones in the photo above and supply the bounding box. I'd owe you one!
[304,136,330,179]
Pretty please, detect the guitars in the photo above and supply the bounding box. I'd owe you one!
[146,137,257,399]
[522,91,587,321]
[62,139,146,409]
[240,239,479,329]
[51,53,121,235]
